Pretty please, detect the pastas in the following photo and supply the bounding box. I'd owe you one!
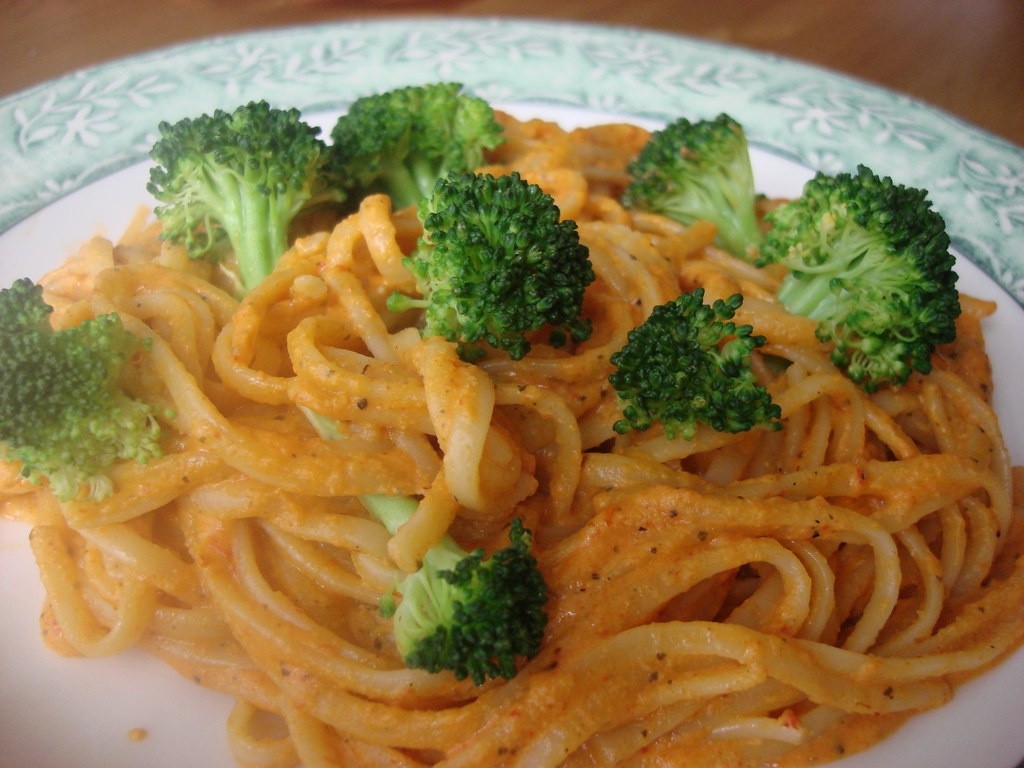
[0,107,1024,767]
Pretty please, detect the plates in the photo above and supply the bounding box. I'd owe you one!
[0,15,1024,767]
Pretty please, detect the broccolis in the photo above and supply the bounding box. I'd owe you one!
[0,83,964,688]
